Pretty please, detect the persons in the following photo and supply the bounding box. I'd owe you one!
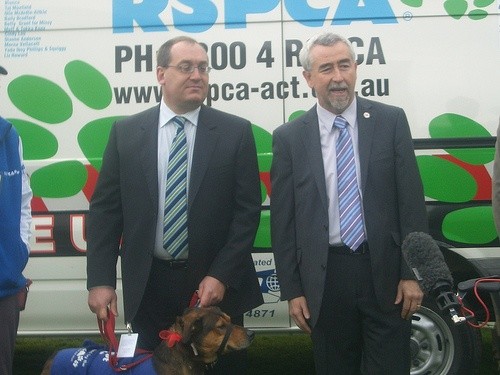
[269,32,430,375]
[85,36,265,375]
[0,117,32,375]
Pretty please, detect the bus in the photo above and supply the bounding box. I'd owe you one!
[0,1,500,375]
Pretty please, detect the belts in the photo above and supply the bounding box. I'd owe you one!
[328,242,369,255]
[154,259,189,270]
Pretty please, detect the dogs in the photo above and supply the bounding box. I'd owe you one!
[41,305,256,375]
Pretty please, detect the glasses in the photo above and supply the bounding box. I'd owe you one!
[163,64,213,73]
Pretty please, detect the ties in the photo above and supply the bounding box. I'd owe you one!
[332,116,366,252]
[163,116,189,261]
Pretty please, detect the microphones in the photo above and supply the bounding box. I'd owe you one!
[401,232,463,314]
